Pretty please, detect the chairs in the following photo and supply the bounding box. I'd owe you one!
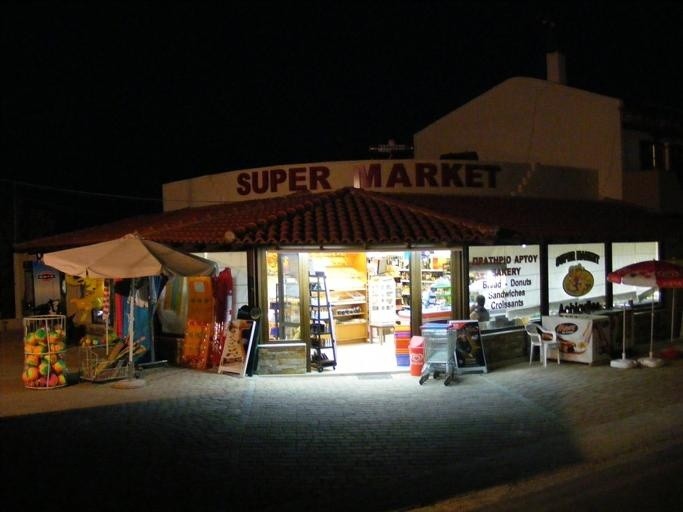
[524,323,562,370]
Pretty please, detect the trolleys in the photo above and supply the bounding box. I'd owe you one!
[419,321,462,387]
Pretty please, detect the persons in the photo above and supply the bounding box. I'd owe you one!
[469,297,490,322]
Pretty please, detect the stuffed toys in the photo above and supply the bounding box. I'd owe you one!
[22,326,66,388]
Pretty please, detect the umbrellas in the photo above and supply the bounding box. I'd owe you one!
[607,257,683,359]
[42,233,219,380]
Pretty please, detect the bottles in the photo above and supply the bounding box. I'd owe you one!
[558,300,603,315]
[582,323,592,344]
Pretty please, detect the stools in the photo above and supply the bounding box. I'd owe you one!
[367,319,396,346]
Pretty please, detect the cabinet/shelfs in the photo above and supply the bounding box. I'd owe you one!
[282,266,369,346]
[392,266,443,310]
[275,271,337,373]
